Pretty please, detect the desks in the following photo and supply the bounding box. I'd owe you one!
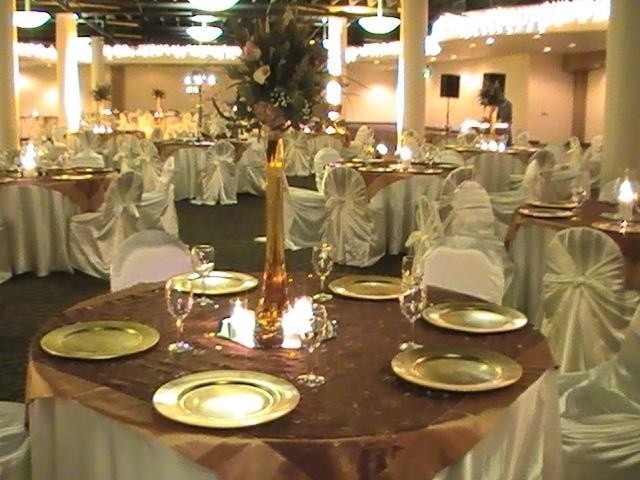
[25,269,558,479]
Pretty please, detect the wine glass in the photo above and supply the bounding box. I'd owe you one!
[163,243,217,356]
[114,111,236,144]
[298,243,333,389]
[3,138,112,180]
[335,128,513,172]
[398,253,426,352]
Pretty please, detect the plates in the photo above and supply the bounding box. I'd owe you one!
[165,268,260,296]
[151,370,303,429]
[391,344,523,394]
[42,320,160,363]
[504,195,638,236]
[328,273,404,300]
[423,301,530,336]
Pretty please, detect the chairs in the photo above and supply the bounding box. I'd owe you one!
[1,402,31,478]
[112,229,194,293]
[139,105,637,333]
[558,305,640,479]
[538,227,638,371]
[1,107,176,280]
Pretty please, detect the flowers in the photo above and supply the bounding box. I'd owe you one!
[207,0,371,195]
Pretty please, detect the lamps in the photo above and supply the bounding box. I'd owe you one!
[13,6,51,30]
[355,0,401,38]
[184,14,224,44]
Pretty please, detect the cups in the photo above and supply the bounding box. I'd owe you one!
[226,294,315,352]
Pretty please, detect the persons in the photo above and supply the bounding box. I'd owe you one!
[475,80,514,148]
